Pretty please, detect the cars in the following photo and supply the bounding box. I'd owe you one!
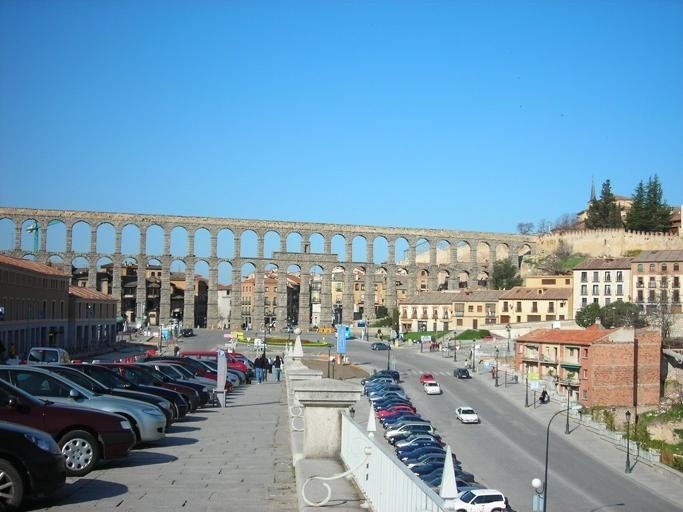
[279,327,293,333]
[327,354,349,363]
[0,365,167,450]
[0,377,137,478]
[360,368,487,497]
[26,350,255,450]
[370,343,391,351]
[182,328,192,337]
[0,419,67,512]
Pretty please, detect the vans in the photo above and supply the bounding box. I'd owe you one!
[26,347,68,366]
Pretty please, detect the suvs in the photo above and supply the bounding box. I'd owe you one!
[441,489,507,512]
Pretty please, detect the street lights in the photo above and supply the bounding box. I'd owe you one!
[326,345,331,378]
[523,364,529,409]
[564,382,571,434]
[158,325,163,352]
[505,322,512,353]
[624,410,631,473]
[529,405,583,512]
[415,322,499,388]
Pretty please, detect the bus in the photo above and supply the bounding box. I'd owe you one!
[335,325,350,340]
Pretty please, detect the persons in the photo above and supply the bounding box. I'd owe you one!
[491,366,496,379]
[253,354,284,384]
[539,390,547,404]
[7,344,18,361]
